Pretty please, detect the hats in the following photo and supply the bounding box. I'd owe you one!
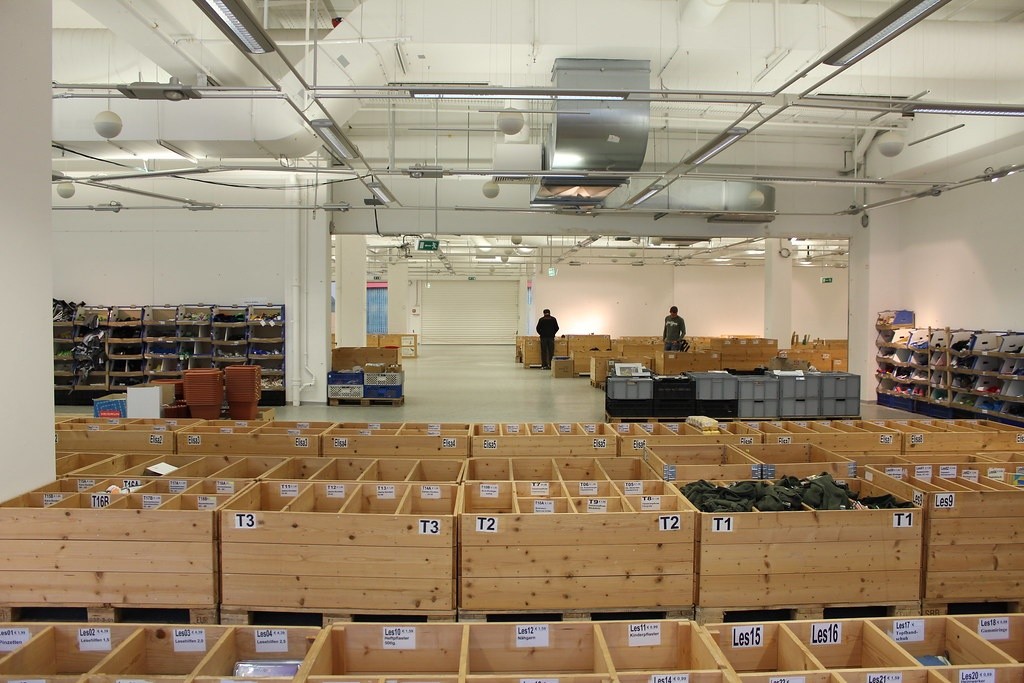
[543,309,550,313]
[670,306,678,312]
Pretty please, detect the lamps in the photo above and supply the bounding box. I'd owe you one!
[57,1,903,276]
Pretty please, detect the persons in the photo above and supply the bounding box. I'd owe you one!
[662,306,686,351]
[536,309,559,370]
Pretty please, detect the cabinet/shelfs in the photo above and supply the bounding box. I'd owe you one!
[55,451,121,479]
[642,443,857,479]
[977,452,1024,473]
[875,309,1024,428]
[845,454,998,477]
[871,418,1023,454]
[296,619,737,683]
[0,479,255,623]
[865,464,1024,613]
[470,422,618,456]
[93,392,127,418]
[0,622,324,682]
[670,478,917,623]
[51,301,287,404]
[740,420,902,454]
[175,420,336,458]
[128,384,162,417]
[701,613,1024,683]
[54,417,202,455]
[322,421,469,458]
[461,456,662,481]
[608,422,759,459]
[67,456,288,479]
[459,481,695,622]
[219,481,459,626]
[260,456,465,484]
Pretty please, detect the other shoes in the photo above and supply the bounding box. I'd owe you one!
[541,365,551,370]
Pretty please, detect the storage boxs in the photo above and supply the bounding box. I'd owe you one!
[516,335,860,415]
[331,334,417,398]
